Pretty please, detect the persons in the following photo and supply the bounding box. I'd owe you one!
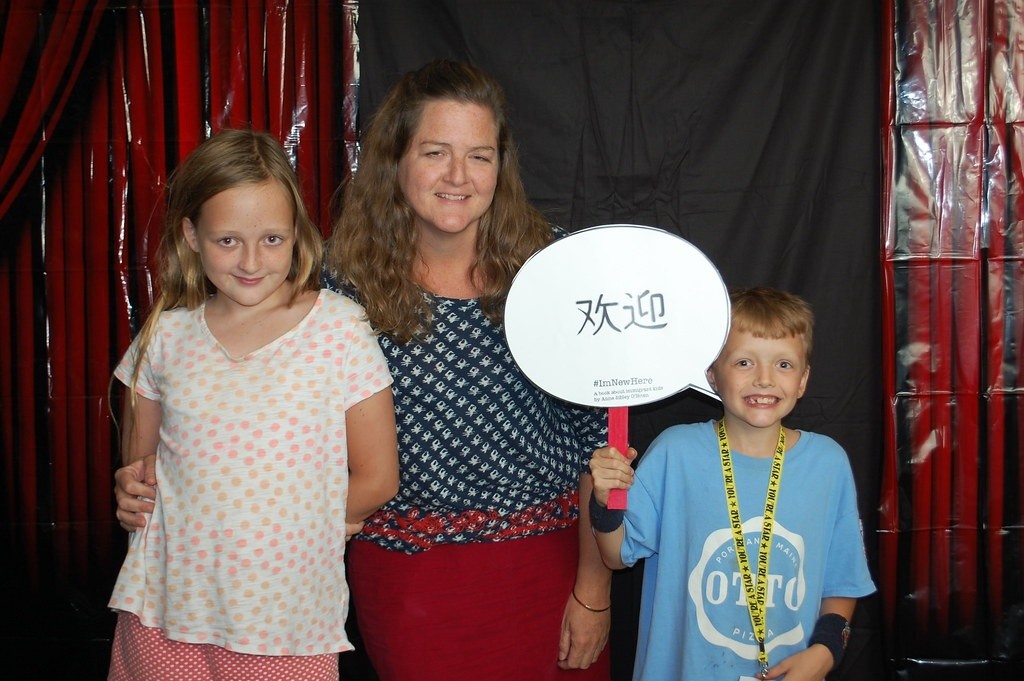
[588,288,876,680]
[115,61,631,680]
[108,129,399,680]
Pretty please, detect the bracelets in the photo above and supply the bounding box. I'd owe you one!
[589,489,626,532]
[572,589,611,612]
[808,612,851,667]
